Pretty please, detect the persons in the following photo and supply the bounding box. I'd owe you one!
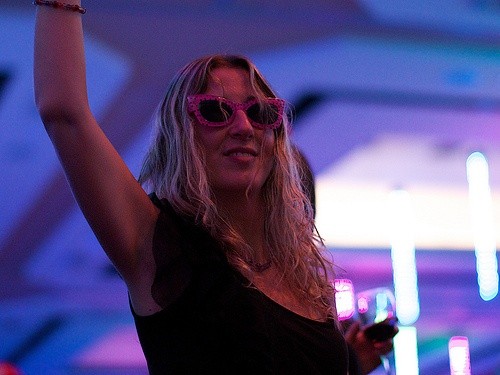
[33,0,400,375]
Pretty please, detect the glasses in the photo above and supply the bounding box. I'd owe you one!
[186,94,285,131]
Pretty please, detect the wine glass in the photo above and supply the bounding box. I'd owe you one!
[355,286,397,375]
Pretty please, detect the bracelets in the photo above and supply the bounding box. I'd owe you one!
[33,0,86,14]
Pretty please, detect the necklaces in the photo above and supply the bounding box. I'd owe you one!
[244,257,272,272]
